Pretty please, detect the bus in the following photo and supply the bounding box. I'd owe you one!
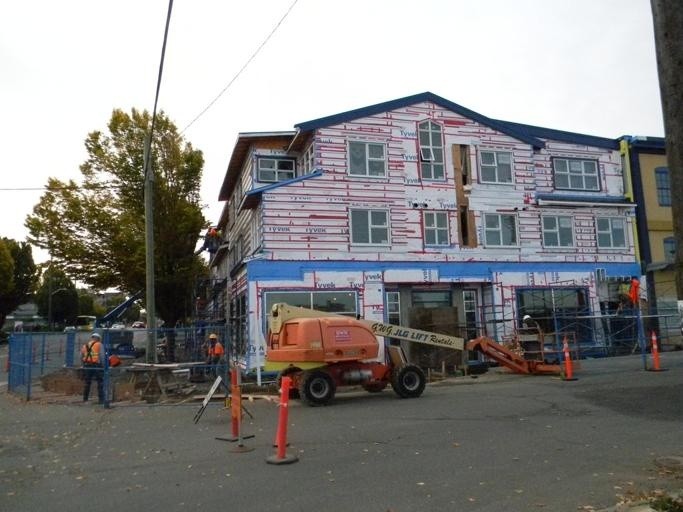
[77,316,96,331]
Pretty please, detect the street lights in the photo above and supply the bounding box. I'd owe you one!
[48,288,66,320]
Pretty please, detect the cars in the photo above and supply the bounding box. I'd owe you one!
[64,326,76,333]
[112,321,145,329]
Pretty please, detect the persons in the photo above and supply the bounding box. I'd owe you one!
[204,332,225,382]
[517,314,541,361]
[79,331,105,404]
[205,226,224,251]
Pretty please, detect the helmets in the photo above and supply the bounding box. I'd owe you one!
[90,332,101,342]
[209,333,217,339]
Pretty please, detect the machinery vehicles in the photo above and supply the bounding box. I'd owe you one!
[265,302,581,407]
[88,234,224,359]
